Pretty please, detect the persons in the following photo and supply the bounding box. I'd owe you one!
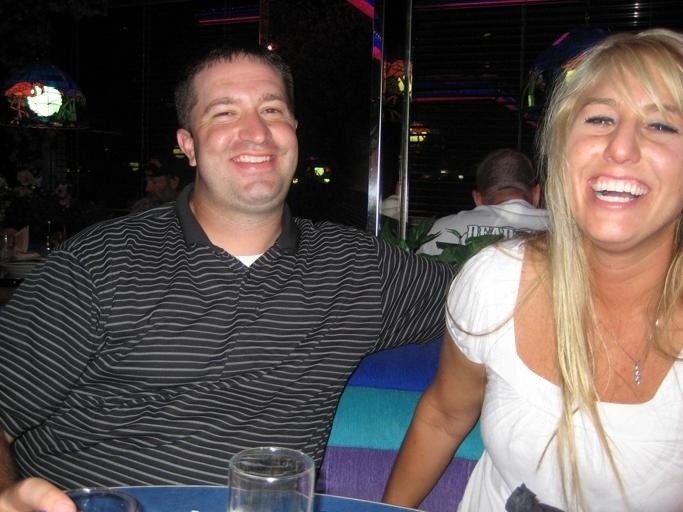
[0,39,460,511]
[413,148,548,257]
[380,28,683,511]
[129,156,182,213]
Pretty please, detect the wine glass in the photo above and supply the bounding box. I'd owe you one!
[0,233,17,261]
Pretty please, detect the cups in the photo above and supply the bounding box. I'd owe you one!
[225,445,317,512]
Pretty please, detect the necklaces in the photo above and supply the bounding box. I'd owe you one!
[594,274,667,386]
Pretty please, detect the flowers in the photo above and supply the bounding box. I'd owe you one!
[0,169,79,234]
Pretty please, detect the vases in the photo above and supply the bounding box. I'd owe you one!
[0,225,28,254]
